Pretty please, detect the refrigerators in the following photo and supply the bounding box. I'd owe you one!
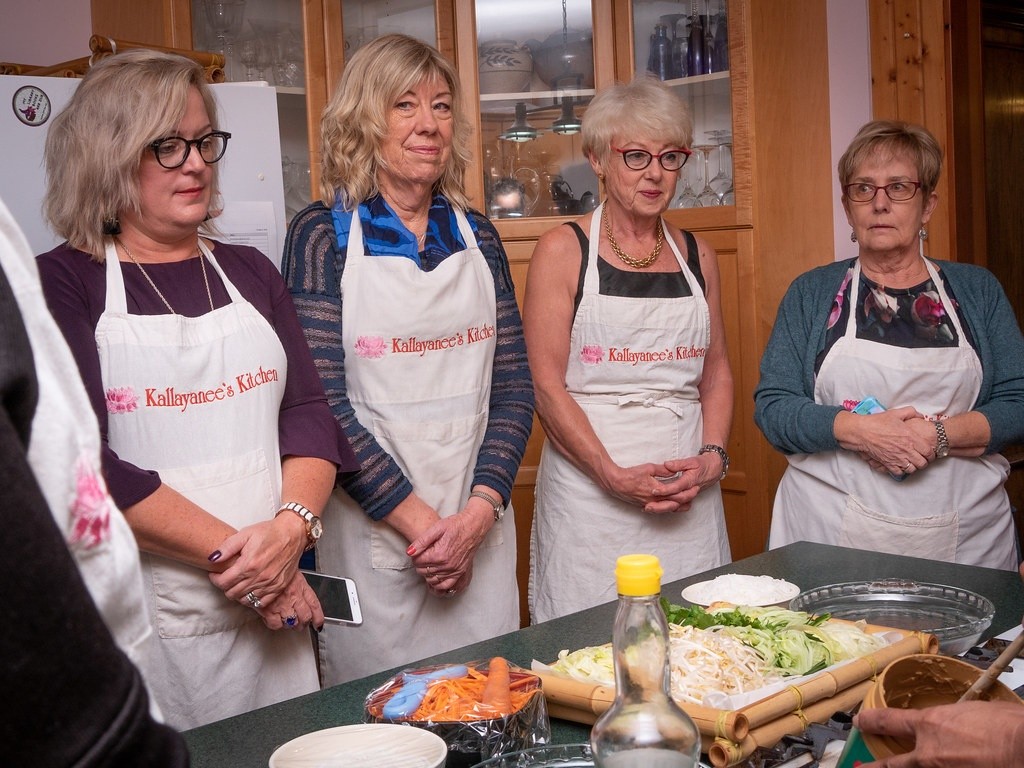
[0,75,288,282]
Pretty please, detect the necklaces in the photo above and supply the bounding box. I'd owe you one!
[602,201,666,268]
[116,234,215,314]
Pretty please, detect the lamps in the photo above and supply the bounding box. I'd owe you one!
[496,102,545,142]
[552,95,582,136]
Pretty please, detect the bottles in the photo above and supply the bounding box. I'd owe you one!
[654,23,672,81]
[675,37,689,78]
[590,554,702,768]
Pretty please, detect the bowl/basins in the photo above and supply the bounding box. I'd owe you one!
[789,578,995,657]
[469,744,592,768]
[681,580,801,610]
[269,724,448,768]
[367,670,543,768]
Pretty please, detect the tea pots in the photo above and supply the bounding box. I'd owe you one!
[484,167,542,218]
[479,24,594,94]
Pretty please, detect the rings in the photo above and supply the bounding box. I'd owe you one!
[427,567,430,577]
[281,612,297,625]
[905,463,911,470]
[246,592,261,608]
[447,589,456,594]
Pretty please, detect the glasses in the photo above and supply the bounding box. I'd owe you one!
[843,181,922,203]
[609,145,691,171]
[144,129,231,169]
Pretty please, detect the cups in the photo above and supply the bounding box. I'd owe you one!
[582,195,599,214]
[834,654,1024,768]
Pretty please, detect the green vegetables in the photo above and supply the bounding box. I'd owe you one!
[661,603,851,672]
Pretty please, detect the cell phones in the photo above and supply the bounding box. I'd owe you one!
[298,568,363,627]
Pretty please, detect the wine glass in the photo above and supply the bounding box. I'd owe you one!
[190,0,404,87]
[282,156,312,226]
[674,130,735,208]
[657,0,730,78]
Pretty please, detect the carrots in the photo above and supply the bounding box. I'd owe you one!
[372,656,536,723]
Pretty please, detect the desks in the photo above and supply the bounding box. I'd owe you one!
[177,541,1024,768]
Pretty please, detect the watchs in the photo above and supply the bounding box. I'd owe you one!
[934,420,950,459]
[468,491,504,522]
[699,445,729,481]
[275,502,322,551]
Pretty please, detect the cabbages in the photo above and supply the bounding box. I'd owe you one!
[552,645,617,687]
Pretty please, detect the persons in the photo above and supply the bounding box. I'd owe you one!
[523,81,733,623]
[281,33,537,688]
[0,195,194,768]
[38,48,357,728]
[751,119,1024,572]
[852,700,1024,768]
